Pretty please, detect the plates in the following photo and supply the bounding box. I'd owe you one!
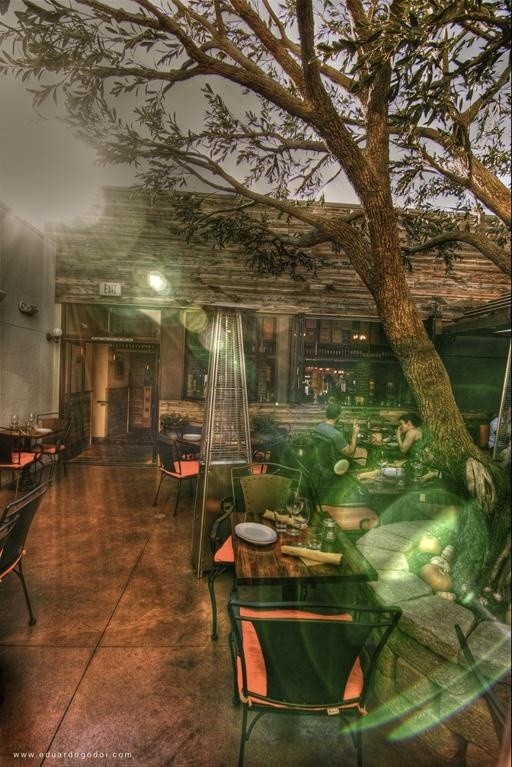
[36,429,52,434]
[235,521,277,545]
[383,468,401,477]
[183,434,202,441]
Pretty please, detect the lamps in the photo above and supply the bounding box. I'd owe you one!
[46,327,63,345]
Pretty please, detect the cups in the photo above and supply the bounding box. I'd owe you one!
[309,528,324,550]
[274,509,289,529]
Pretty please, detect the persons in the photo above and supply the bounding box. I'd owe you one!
[315,402,365,471]
[387,412,424,469]
[486,403,512,460]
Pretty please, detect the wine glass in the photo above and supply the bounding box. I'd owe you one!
[286,488,311,548]
[11,412,35,433]
[375,451,388,482]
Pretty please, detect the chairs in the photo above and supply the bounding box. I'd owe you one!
[0,412,73,626]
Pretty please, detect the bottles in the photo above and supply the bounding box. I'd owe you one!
[322,518,337,543]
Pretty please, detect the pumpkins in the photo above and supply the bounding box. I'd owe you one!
[419,563,452,590]
[441,545,456,562]
[430,555,450,572]
[436,591,456,602]
[418,535,440,555]
